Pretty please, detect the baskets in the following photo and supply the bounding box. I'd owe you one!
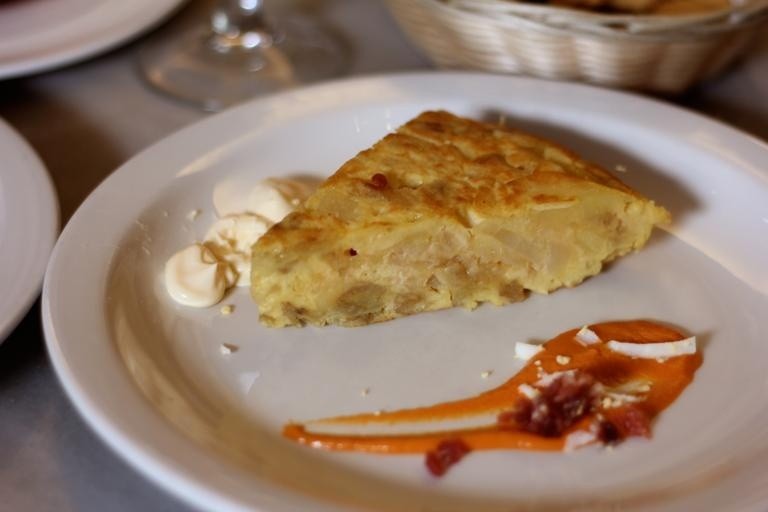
[383,0,768,90]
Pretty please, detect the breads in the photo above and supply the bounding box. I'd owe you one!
[250,112,668,327]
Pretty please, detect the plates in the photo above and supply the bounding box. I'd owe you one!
[44,71,766,510]
[2,0,184,78]
[0,118,59,338]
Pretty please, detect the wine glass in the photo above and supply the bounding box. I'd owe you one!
[135,0,349,109]
